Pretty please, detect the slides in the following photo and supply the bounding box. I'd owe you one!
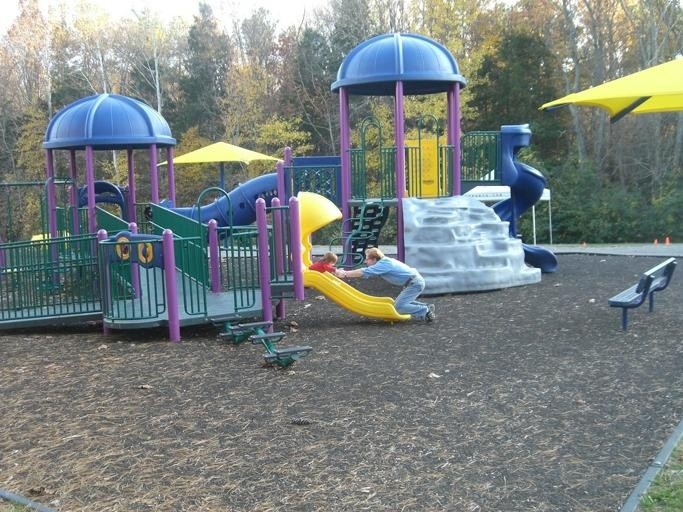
[492,125,556,272]
[303,264,410,321]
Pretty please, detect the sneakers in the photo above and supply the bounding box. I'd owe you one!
[426,304,435,321]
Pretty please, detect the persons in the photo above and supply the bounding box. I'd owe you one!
[307,251,340,274]
[335,248,436,323]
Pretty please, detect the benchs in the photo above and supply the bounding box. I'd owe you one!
[608,257,676,329]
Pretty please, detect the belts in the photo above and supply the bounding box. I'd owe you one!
[405,275,415,286]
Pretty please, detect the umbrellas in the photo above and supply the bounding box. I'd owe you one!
[156,141,282,248]
[538,56,683,123]
